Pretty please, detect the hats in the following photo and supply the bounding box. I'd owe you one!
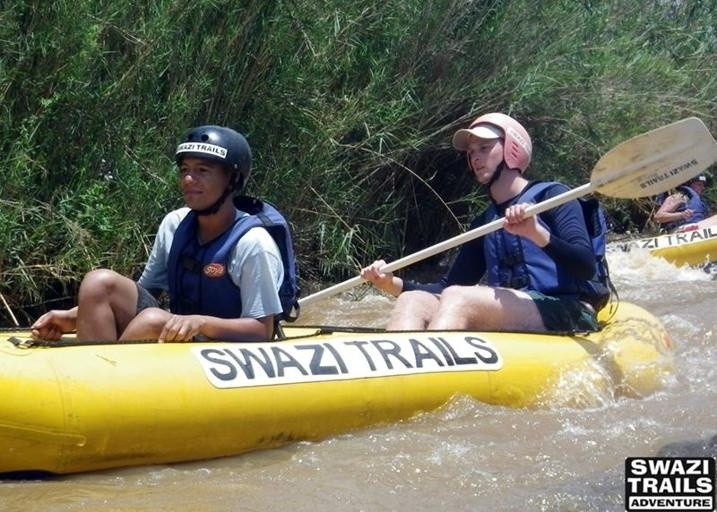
[451,122,505,152]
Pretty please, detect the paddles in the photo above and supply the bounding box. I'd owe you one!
[297,117,717,307]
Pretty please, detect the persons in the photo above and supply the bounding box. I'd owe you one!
[27,124,287,344]
[351,110,597,337]
[650,170,715,236]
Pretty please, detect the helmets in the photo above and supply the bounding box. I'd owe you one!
[686,172,708,185]
[466,111,534,175]
[175,125,253,192]
